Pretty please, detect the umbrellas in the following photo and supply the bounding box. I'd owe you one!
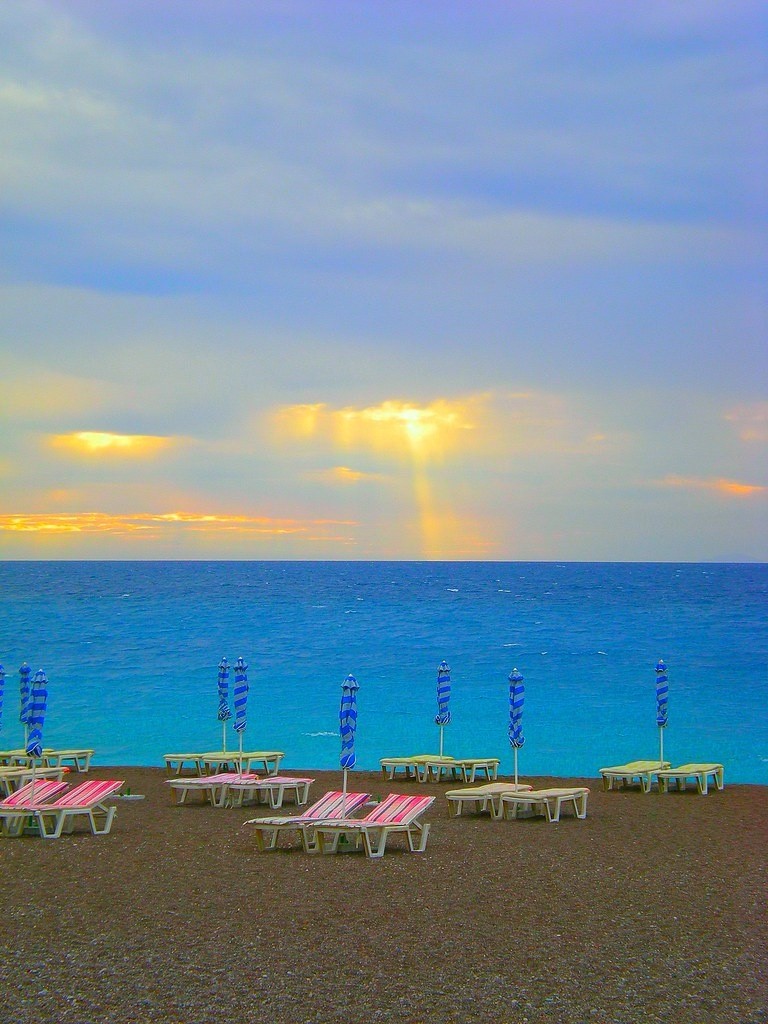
[0,665,6,732]
[339,674,360,819]
[435,661,452,759]
[507,668,525,792]
[19,662,32,748]
[25,669,49,806]
[233,657,249,773]
[655,660,669,770]
[217,657,232,754]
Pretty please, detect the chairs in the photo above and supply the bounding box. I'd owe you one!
[380,755,500,783]
[444,782,532,821]
[242,791,372,854]
[598,760,671,793]
[202,751,284,777]
[162,752,243,778]
[501,787,590,823]
[222,776,315,810]
[654,764,725,795]
[163,773,258,808]
[310,793,436,858]
[0,748,125,838]
